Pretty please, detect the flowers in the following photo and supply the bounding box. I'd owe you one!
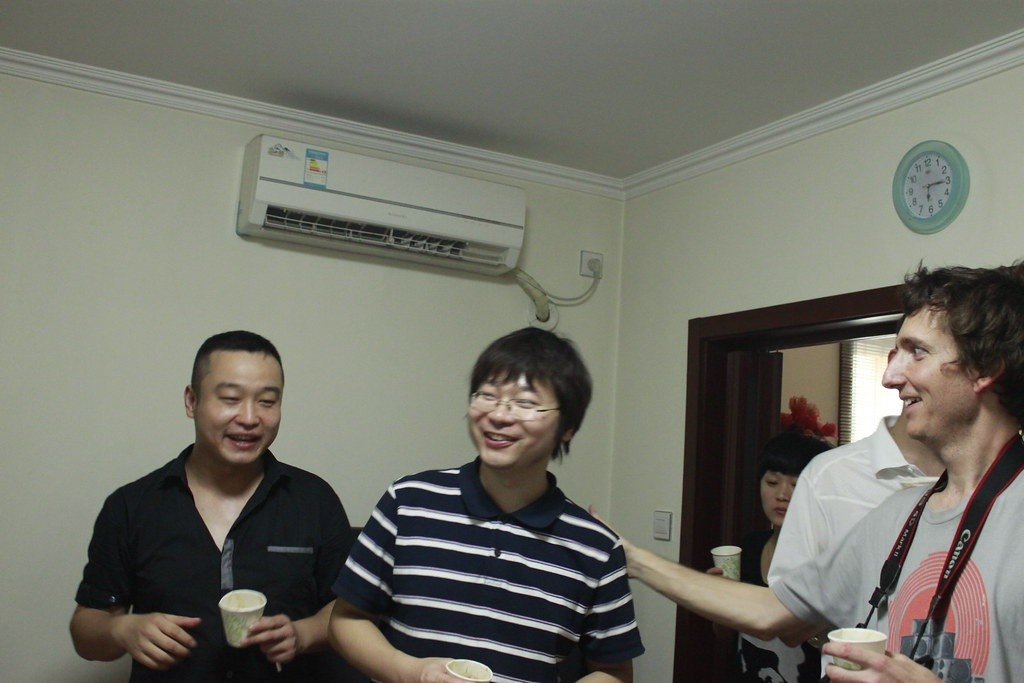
[780,395,837,437]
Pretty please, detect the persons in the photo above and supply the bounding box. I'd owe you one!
[327,326,644,683]
[768,349,944,683]
[587,261,1024,683]
[707,429,832,683]
[68,330,352,683]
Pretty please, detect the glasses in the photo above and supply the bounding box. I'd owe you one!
[471,391,562,420]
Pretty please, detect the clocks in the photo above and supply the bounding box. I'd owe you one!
[892,139,970,234]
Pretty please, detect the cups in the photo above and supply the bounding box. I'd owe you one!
[218,589,267,647]
[828,628,889,671]
[711,546,742,581]
[445,659,493,683]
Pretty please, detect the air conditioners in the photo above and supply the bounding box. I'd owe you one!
[235,134,527,278]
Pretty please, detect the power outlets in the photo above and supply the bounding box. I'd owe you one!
[579,250,604,279]
